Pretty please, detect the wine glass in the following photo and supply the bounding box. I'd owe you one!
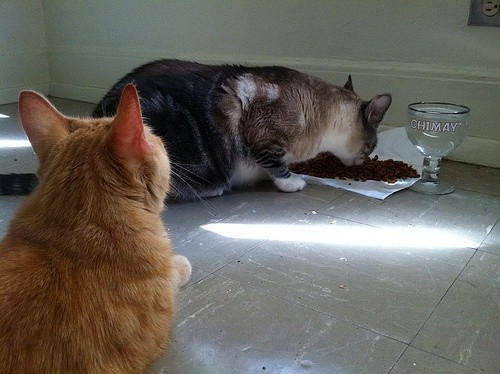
[406,102,470,194]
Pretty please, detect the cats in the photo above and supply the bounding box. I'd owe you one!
[0,57,393,200]
[1,84,194,374]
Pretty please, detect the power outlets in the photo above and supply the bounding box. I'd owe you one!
[467,0,500,26]
